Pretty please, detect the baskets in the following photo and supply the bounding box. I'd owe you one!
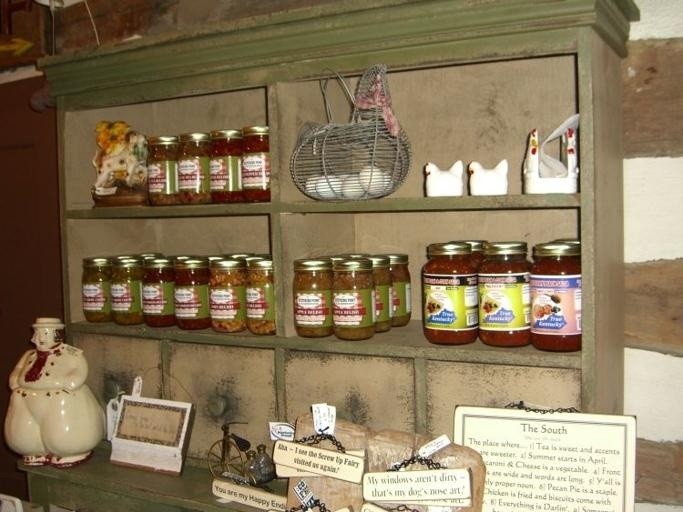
[290,61,412,203]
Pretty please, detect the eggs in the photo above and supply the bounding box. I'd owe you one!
[303,165,393,202]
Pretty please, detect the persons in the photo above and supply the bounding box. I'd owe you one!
[6,316,108,469]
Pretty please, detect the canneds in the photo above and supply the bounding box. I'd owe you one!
[419,238,581,353]
[146,124,271,206]
[81,250,276,336]
[292,252,413,341]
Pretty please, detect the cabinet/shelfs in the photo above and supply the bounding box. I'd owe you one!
[270,0,641,348]
[63,331,278,474]
[33,11,277,341]
[280,348,621,446]
[16,448,286,512]
